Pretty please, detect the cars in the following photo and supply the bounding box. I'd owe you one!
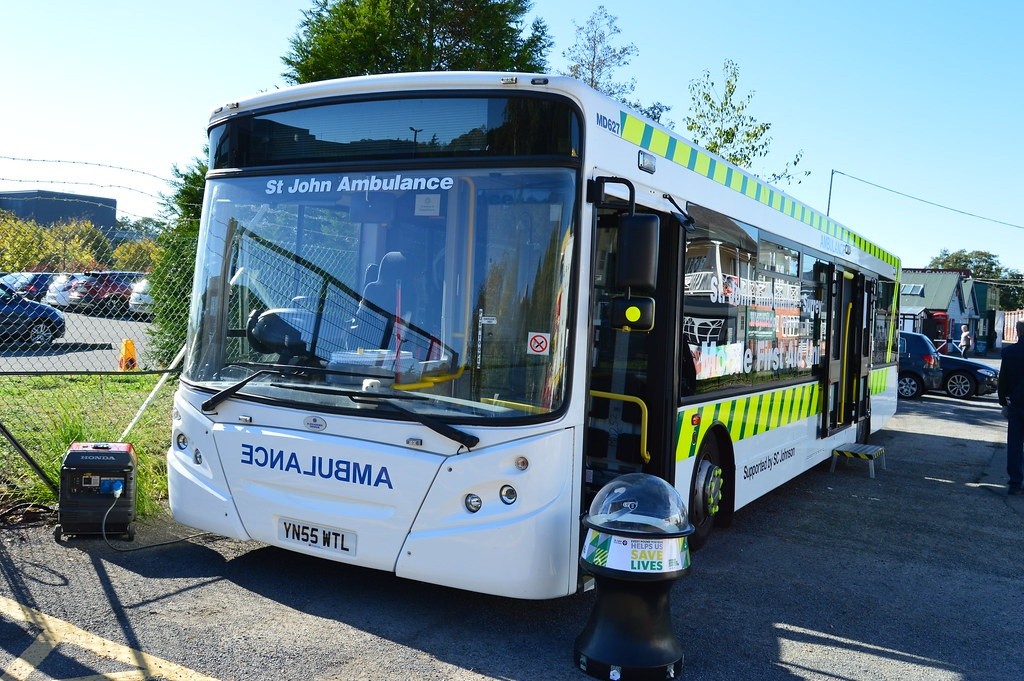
[938,354,1000,398]
[0,279,65,347]
[0,273,195,318]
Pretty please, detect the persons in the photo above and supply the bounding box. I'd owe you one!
[998,320,1024,495]
[957,325,973,359]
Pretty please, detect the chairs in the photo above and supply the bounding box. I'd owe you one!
[343,249,433,361]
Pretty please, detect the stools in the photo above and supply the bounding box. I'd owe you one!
[829,442,888,478]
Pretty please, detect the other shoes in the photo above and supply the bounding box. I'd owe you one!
[1007,480,1024,493]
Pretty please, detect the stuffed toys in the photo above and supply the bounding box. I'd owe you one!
[357,379,380,409]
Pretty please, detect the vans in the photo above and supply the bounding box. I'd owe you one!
[898,330,939,401]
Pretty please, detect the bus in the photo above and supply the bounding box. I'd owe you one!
[166,66,898,614]
